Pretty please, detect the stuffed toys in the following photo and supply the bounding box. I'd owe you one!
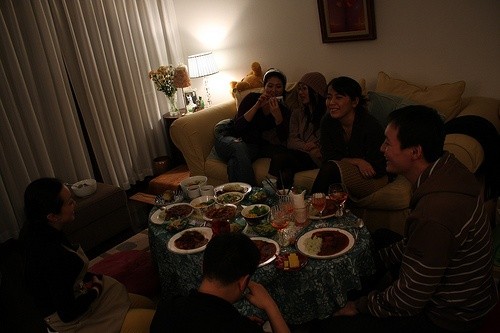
[230,63,266,98]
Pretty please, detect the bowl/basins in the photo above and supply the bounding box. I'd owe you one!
[190,195,216,208]
[71,179,97,197]
[162,216,189,233]
[245,188,270,203]
[180,176,208,192]
[241,204,271,226]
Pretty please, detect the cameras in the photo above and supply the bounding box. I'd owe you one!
[270,96,284,104]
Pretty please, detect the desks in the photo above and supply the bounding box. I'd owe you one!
[163,108,205,169]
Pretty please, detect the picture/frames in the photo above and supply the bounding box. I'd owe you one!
[317,0,377,44]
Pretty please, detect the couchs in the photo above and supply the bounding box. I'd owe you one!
[170,83,500,236]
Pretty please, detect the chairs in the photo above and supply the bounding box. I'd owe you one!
[0,238,40,333]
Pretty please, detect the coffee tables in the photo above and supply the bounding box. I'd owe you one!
[148,187,374,333]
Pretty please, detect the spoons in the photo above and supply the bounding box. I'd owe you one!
[354,218,365,242]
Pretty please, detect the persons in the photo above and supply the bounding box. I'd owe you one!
[150,229,290,333]
[310,106,495,333]
[269,72,327,189]
[309,77,397,200]
[214,66,287,188]
[1,179,132,333]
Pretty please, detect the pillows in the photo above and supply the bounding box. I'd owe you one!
[367,71,469,133]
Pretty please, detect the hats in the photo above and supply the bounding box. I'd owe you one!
[298,72,327,97]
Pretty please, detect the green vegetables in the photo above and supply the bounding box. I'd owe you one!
[202,199,214,205]
[257,220,276,234]
[223,194,231,202]
[248,205,268,216]
[248,190,267,201]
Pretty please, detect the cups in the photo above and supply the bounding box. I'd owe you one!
[262,179,277,192]
[276,189,292,205]
[187,185,200,198]
[292,203,310,226]
[200,185,214,196]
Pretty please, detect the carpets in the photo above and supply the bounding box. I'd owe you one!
[86,227,159,299]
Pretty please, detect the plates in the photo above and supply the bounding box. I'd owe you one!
[306,196,344,219]
[214,182,252,195]
[216,192,244,205]
[250,237,280,268]
[275,253,308,272]
[203,204,237,221]
[155,191,185,205]
[167,227,213,255]
[150,203,194,225]
[297,228,355,259]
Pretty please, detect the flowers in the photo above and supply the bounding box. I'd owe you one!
[149,64,177,98]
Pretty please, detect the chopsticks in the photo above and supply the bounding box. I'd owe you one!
[264,175,282,195]
[282,184,292,195]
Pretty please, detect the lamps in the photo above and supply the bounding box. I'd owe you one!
[187,52,223,109]
[174,66,192,116]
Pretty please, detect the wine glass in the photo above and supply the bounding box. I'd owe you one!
[270,205,292,252]
[328,183,352,230]
[312,193,330,228]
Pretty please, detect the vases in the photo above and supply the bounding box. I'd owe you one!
[163,91,180,117]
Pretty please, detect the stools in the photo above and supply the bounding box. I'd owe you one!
[59,182,135,261]
